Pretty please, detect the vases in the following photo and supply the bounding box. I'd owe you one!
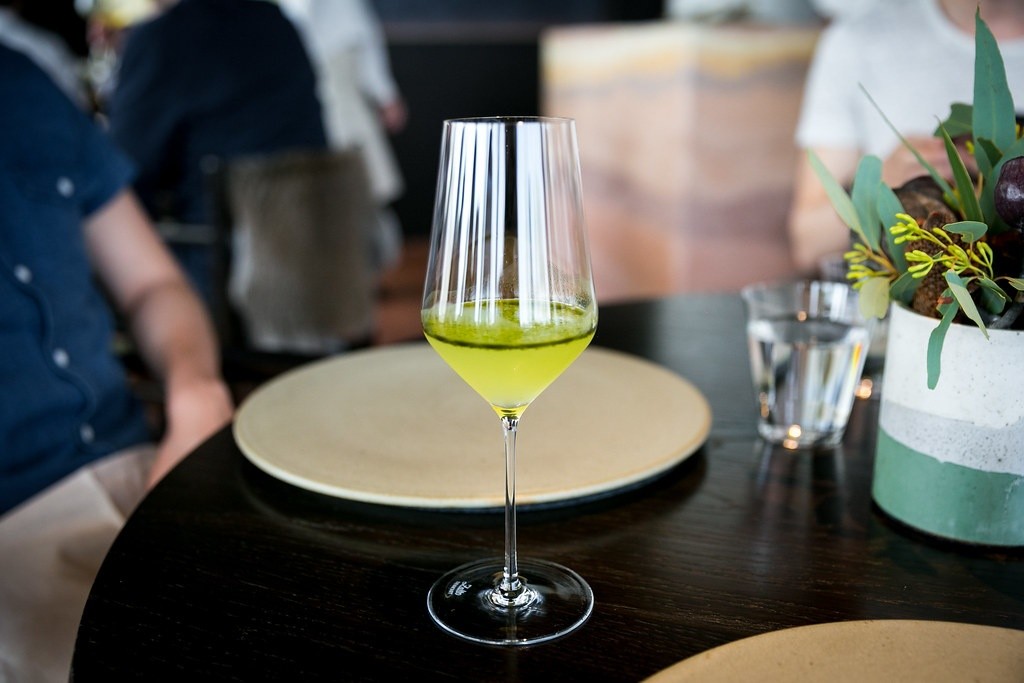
[874,302,1023,547]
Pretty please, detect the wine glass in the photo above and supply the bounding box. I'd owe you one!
[420,114,598,647]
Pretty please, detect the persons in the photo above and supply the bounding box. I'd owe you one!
[788,1,1024,272]
[1,0,408,683]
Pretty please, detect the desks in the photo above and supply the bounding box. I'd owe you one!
[66,276,1024,683]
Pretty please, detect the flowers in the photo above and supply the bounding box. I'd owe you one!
[810,0,1024,388]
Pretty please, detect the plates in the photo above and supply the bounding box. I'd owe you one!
[637,618,1024,683]
[231,342,713,514]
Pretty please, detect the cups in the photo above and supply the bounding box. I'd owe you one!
[739,278,878,452]
